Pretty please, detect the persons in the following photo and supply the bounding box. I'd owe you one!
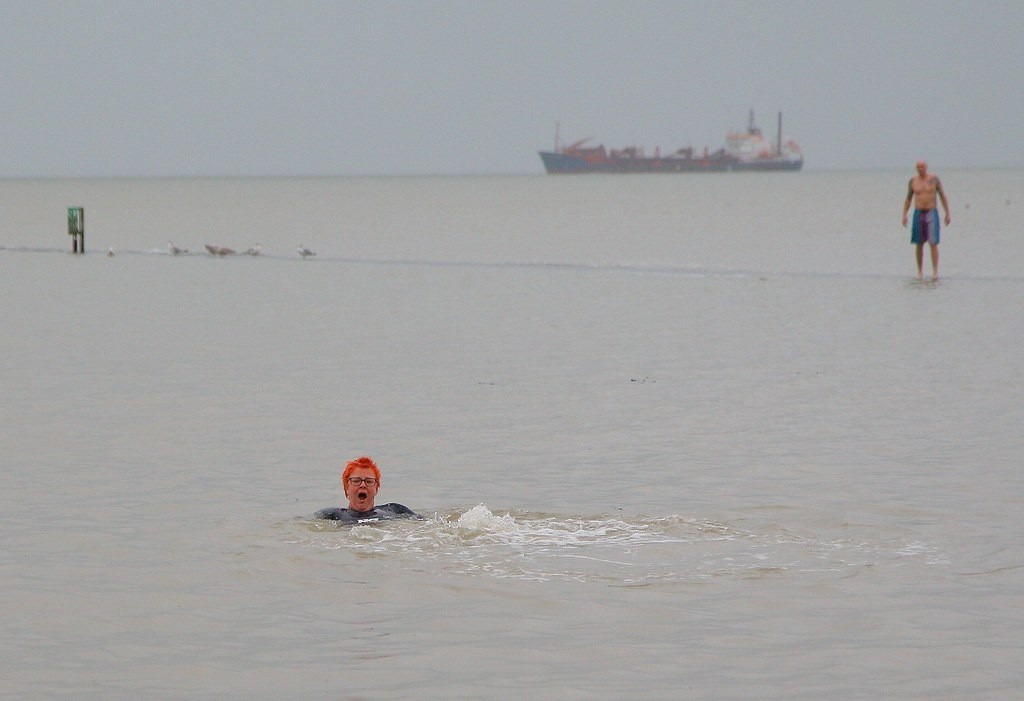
[902,161,951,279]
[317,458,415,522]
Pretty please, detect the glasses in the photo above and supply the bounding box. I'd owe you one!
[347,476,378,487]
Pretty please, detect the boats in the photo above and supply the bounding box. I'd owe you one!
[538,106,805,173]
